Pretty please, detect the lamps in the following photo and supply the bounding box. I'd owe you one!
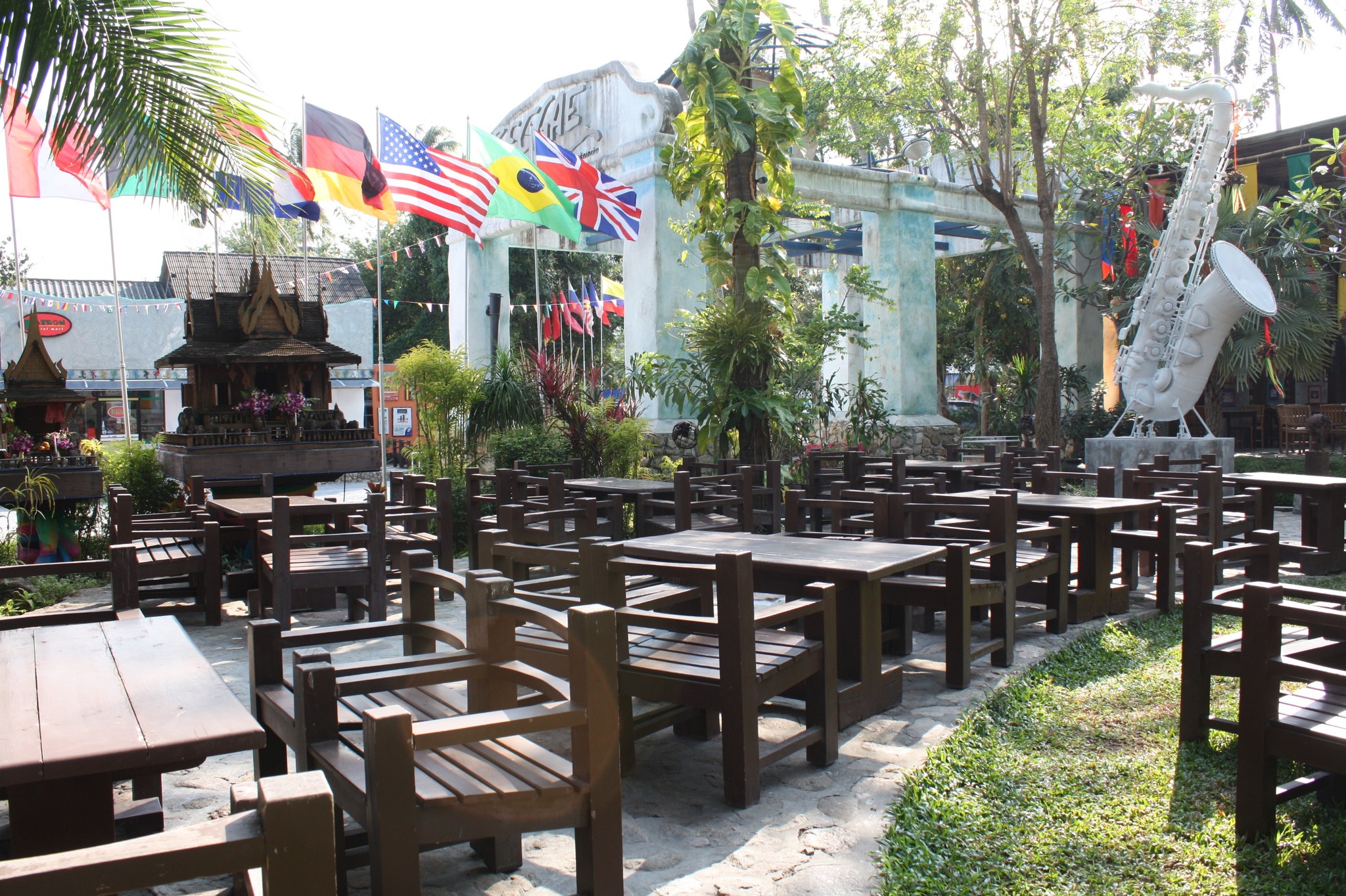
[902,137,931,175]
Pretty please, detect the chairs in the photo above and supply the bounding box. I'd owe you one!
[0,403,1346,895]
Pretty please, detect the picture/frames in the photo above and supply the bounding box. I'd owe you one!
[1324,367,1328,382]
[1310,357,1320,381]
[1308,385,1321,404]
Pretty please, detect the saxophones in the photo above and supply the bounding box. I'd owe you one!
[1115,82,1278,420]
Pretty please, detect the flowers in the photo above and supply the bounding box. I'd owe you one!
[234,390,319,420]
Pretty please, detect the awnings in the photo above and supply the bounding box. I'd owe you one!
[0,379,381,390]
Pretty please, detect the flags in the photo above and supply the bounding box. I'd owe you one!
[48,103,111,210]
[0,232,624,313]
[468,121,582,242]
[602,276,624,317]
[543,301,552,346]
[589,277,610,325]
[582,283,594,337]
[569,279,583,319]
[0,78,42,199]
[302,100,398,226]
[105,97,205,202]
[378,112,502,251]
[552,293,561,342]
[533,129,641,243]
[559,286,583,336]
[213,97,320,224]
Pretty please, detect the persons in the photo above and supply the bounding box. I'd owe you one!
[86,419,96,441]
